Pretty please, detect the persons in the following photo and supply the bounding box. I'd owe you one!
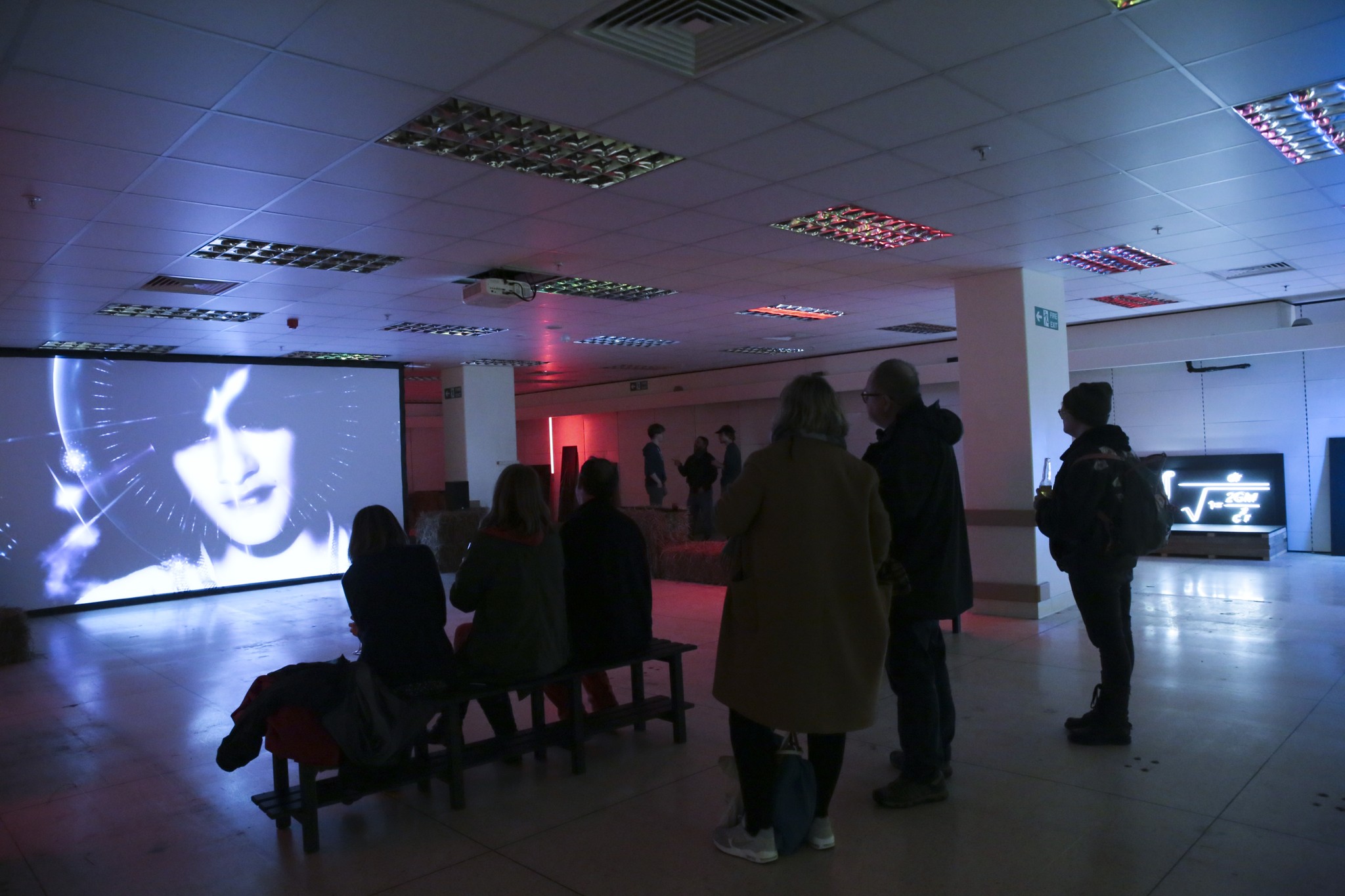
[858,355,974,818]
[640,422,671,507]
[323,502,456,804]
[716,422,743,486]
[698,368,894,869]
[1025,380,1147,748]
[446,463,570,768]
[669,433,718,545]
[555,456,656,718]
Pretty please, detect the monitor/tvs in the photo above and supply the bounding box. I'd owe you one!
[1144,452,1289,528]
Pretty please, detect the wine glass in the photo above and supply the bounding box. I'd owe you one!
[353,621,363,655]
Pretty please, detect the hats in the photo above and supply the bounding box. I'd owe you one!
[1063,381,1112,427]
[715,425,735,434]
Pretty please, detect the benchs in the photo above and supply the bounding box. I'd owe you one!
[263,635,702,851]
[1165,523,1287,561]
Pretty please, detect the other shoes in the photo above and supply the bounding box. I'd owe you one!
[426,726,464,746]
[502,754,524,766]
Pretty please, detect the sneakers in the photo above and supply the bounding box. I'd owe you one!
[713,822,778,864]
[806,815,835,849]
[871,769,949,808]
[889,750,953,777]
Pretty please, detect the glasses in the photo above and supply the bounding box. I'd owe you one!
[1058,409,1070,419]
[861,389,895,404]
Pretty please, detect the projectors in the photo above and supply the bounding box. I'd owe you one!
[461,277,534,309]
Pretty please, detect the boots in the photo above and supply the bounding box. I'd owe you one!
[1064,670,1108,733]
[1067,678,1132,745]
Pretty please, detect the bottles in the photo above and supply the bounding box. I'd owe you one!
[1039,458,1053,498]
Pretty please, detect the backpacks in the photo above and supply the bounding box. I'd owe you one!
[1062,446,1175,557]
